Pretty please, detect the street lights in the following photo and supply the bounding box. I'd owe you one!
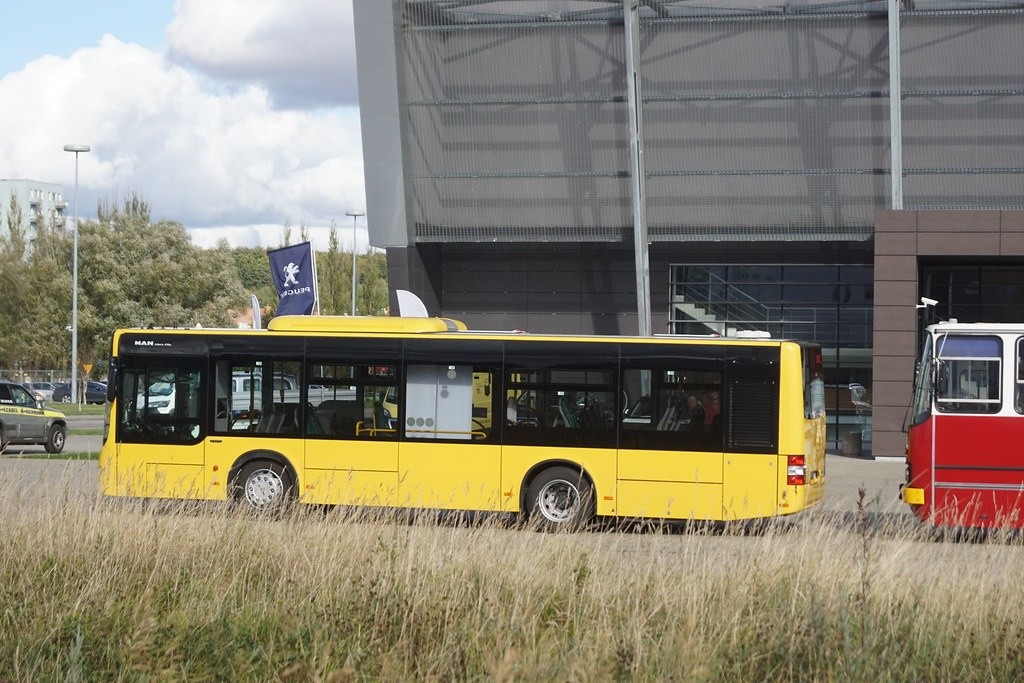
[64,144,93,404]
[344,210,366,316]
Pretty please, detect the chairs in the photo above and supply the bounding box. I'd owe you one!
[521,391,686,432]
[258,398,366,439]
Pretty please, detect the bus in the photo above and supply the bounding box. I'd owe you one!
[900,318,1024,545]
[98,314,828,535]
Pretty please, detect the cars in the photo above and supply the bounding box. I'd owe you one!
[470,368,667,427]
[0,379,69,455]
[12,370,401,441]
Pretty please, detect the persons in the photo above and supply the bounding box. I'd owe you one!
[685,390,721,426]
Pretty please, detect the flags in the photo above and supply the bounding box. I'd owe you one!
[269,241,313,316]
